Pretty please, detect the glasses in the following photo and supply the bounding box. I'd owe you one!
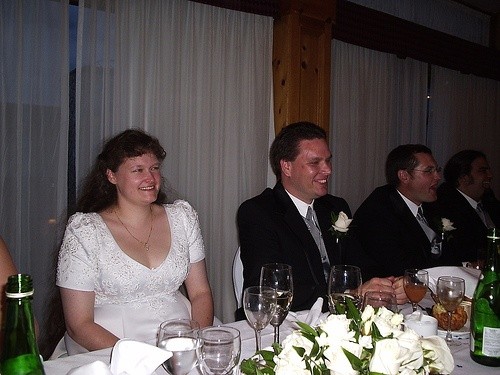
[412,163,440,175]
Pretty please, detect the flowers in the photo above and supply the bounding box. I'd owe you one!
[441,216,456,235]
[328,211,353,241]
[231,295,455,375]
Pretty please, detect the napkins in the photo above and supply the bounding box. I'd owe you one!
[280,297,330,330]
[67,338,173,374]
[416,266,484,300]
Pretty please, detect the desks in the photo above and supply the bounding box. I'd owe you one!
[45,289,500,375]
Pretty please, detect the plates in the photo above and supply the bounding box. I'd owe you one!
[400,306,470,334]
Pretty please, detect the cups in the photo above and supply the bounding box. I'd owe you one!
[363,291,399,313]
[195,324,241,375]
[158,319,200,375]
[327,264,363,315]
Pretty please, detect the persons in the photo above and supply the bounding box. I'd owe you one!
[0,238,39,341]
[421,150,500,270]
[352,143,479,283]
[235,121,412,321]
[56,127,224,357]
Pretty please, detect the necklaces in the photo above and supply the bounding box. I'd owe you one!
[113,206,153,252]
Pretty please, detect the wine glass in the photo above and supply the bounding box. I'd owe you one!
[436,276,466,347]
[403,268,429,312]
[242,286,278,352]
[260,262,294,343]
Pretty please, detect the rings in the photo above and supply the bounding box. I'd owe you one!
[379,287,382,293]
[378,295,381,301]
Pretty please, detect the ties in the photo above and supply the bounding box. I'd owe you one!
[306,205,315,227]
[475,202,494,231]
[416,205,429,227]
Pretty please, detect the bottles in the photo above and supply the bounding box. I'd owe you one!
[0,274,46,375]
[470,227,500,367]
